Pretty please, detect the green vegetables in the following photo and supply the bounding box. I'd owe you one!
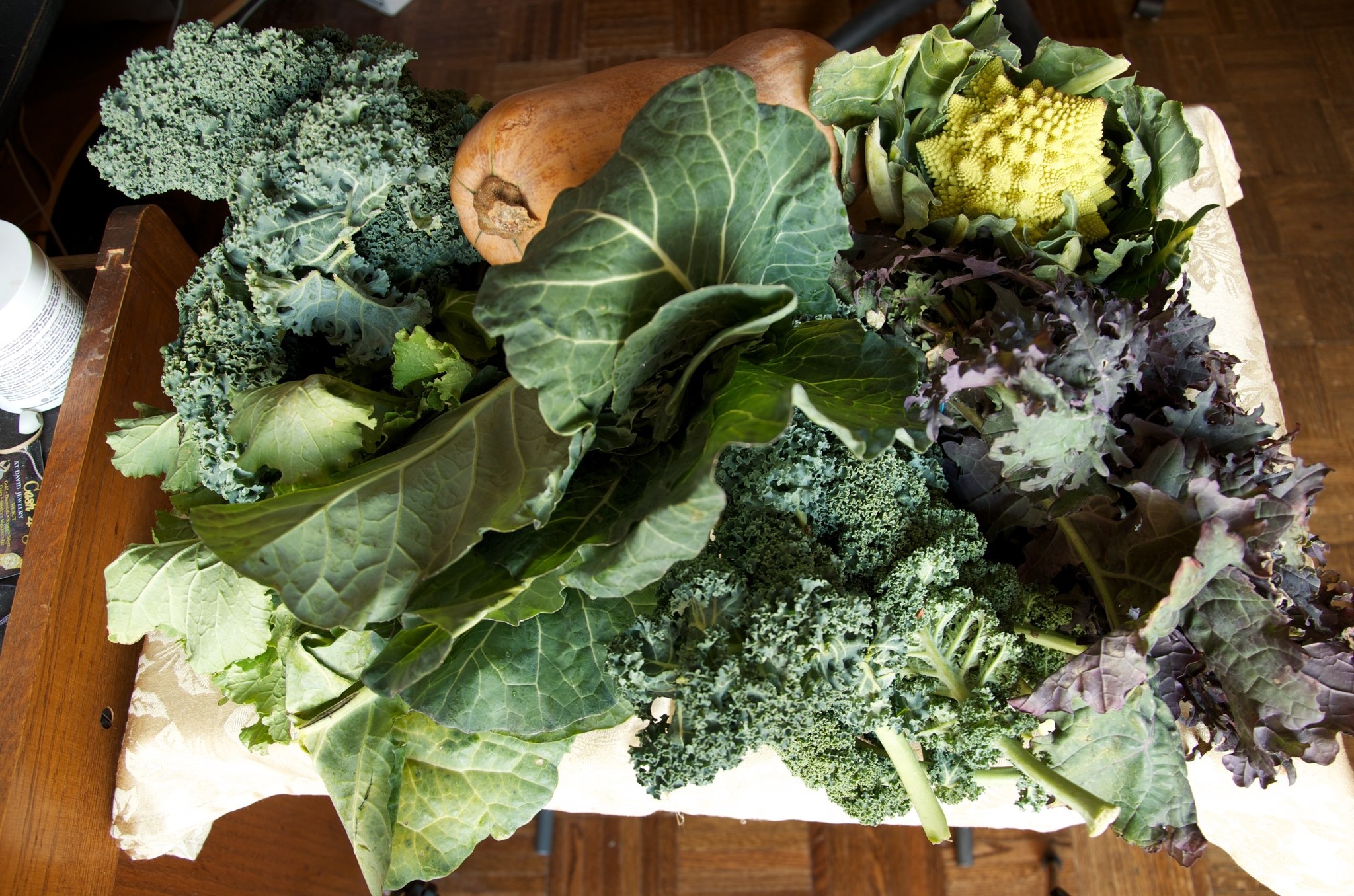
[76,16,1353,895]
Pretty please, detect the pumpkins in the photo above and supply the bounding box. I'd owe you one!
[453,28,847,267]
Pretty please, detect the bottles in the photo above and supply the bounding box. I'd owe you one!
[0,220,88,414]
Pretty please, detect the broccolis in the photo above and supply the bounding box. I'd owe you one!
[913,54,1116,241]
